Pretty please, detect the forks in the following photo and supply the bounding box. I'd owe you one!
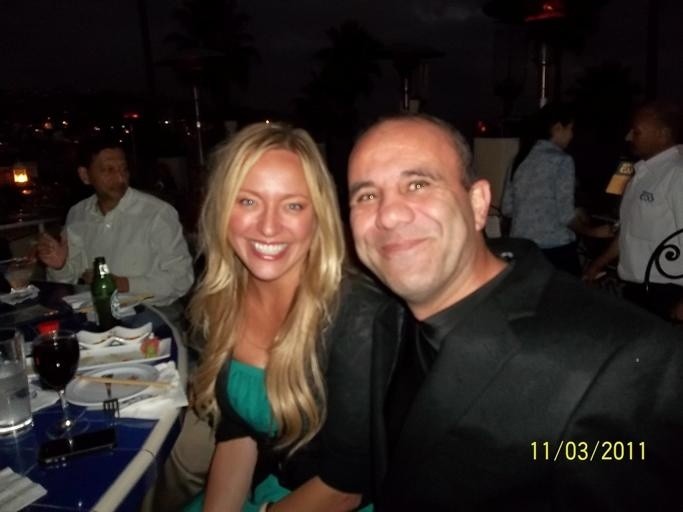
[100,375,122,436]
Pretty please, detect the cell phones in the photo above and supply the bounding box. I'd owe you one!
[38,428,116,466]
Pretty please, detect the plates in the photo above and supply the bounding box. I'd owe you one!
[71,322,155,348]
[1,356,60,415]
[61,337,173,372]
[23,341,33,358]
[86,294,144,314]
[63,363,156,408]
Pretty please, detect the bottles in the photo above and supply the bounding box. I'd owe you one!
[610,219,619,234]
[144,330,161,359]
[90,255,122,331]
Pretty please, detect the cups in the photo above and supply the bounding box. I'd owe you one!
[1,332,37,440]
[43,222,69,251]
[2,256,37,293]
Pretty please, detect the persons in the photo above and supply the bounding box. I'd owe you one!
[39,130,195,334]
[501,101,617,272]
[179,123,387,512]
[348,114,683,512]
[582,102,683,332]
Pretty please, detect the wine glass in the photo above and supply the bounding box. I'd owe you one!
[30,331,91,440]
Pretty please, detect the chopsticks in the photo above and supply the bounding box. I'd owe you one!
[77,371,175,390]
